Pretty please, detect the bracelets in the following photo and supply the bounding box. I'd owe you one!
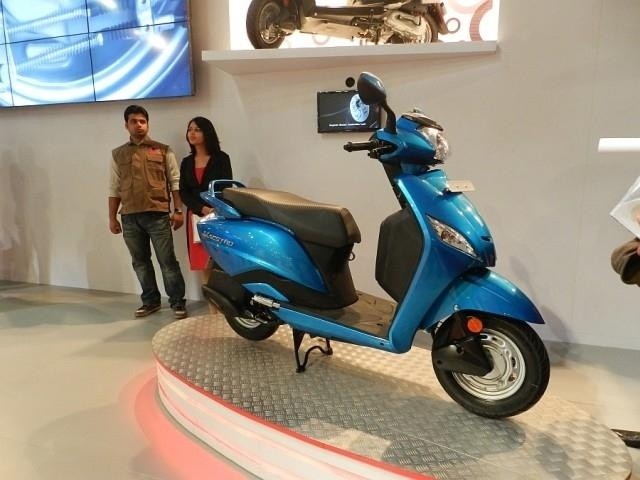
[173,208,184,215]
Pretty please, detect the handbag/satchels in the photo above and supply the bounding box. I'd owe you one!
[191,210,219,245]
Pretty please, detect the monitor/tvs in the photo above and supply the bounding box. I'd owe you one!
[317,91,381,133]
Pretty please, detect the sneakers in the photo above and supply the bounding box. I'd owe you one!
[135,302,161,318]
[172,297,187,320]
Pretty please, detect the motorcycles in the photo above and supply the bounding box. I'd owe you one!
[196,71,552,421]
[246,0,453,49]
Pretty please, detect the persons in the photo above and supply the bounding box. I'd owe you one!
[178,115,233,314]
[106,103,188,320]
